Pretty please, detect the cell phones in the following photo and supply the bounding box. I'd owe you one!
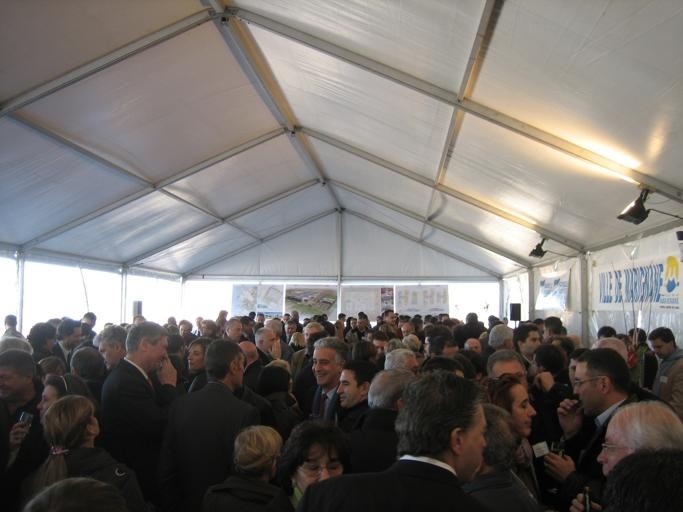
[583,487,590,512]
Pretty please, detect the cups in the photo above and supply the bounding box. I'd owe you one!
[16,411,31,438]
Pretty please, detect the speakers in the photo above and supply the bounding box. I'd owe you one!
[132,300,142,316]
[510,303,521,321]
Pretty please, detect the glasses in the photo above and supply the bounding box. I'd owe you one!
[602,443,630,456]
[574,376,605,386]
[298,464,343,476]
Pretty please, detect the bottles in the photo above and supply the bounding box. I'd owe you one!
[582,486,592,511]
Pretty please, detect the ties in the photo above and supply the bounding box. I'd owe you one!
[318,393,327,417]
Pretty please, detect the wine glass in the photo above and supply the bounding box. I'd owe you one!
[542,442,566,493]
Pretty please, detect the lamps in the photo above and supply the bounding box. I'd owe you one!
[617,187,651,226]
[529,237,548,258]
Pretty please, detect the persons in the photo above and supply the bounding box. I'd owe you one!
[0,309,683,512]
